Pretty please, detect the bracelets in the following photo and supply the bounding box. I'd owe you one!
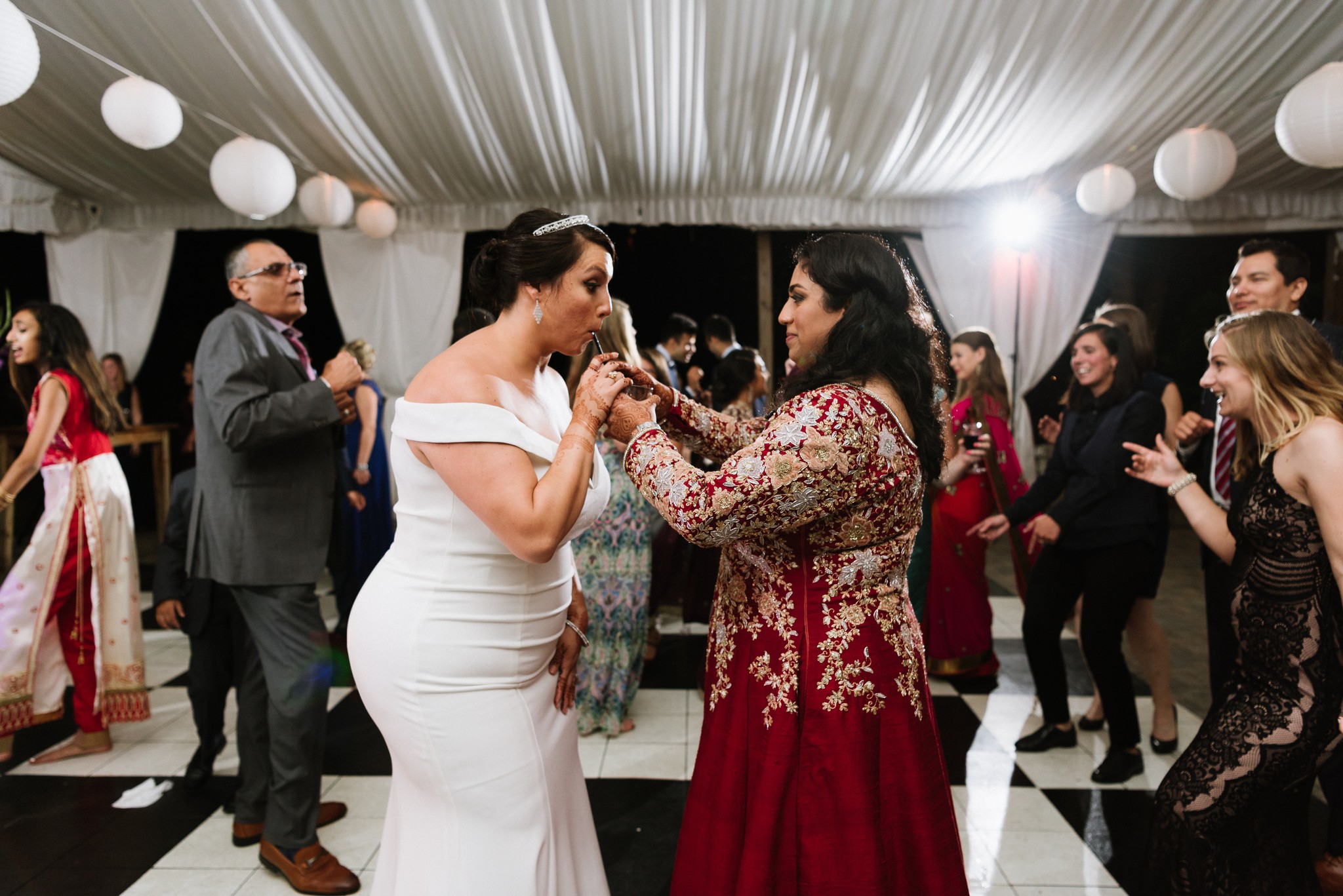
[565,619,590,648]
[355,463,369,473]
[0,486,16,511]
[1167,471,1198,496]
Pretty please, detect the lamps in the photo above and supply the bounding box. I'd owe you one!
[355,200,400,238]
[1273,60,1343,170]
[1076,164,1137,217]
[1,0,42,106]
[209,135,297,220]
[99,76,184,150]
[298,173,355,227]
[1152,126,1238,203]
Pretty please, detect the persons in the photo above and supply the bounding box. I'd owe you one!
[1174,237,1314,711]
[313,416,364,651]
[185,236,368,896]
[703,312,766,425]
[344,207,632,896]
[962,323,1165,785]
[178,360,198,473]
[329,333,393,649]
[928,328,1013,695]
[94,349,155,538]
[623,344,683,676]
[1125,312,1342,896]
[604,230,975,896]
[1036,302,1184,756]
[0,303,155,764]
[153,460,254,814]
[684,348,771,624]
[559,298,658,738]
[637,312,704,410]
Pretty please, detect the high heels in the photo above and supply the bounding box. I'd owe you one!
[1150,703,1178,754]
[1078,715,1105,731]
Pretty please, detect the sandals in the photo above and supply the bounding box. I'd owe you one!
[0,735,14,754]
[28,728,114,767]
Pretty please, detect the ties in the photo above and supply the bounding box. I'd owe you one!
[1213,414,1238,502]
[282,328,316,382]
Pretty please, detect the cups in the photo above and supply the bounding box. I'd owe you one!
[624,386,654,404]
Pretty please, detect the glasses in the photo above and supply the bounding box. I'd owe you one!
[237,262,308,280]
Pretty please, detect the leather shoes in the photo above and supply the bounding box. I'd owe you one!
[1091,747,1145,784]
[185,733,227,788]
[223,775,241,814]
[232,801,348,847]
[259,831,361,896]
[1015,723,1077,750]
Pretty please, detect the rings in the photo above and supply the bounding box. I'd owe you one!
[343,408,351,416]
[607,370,618,382]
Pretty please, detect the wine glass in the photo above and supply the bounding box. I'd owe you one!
[961,420,989,475]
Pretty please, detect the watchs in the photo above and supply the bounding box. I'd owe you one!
[628,421,662,445]
